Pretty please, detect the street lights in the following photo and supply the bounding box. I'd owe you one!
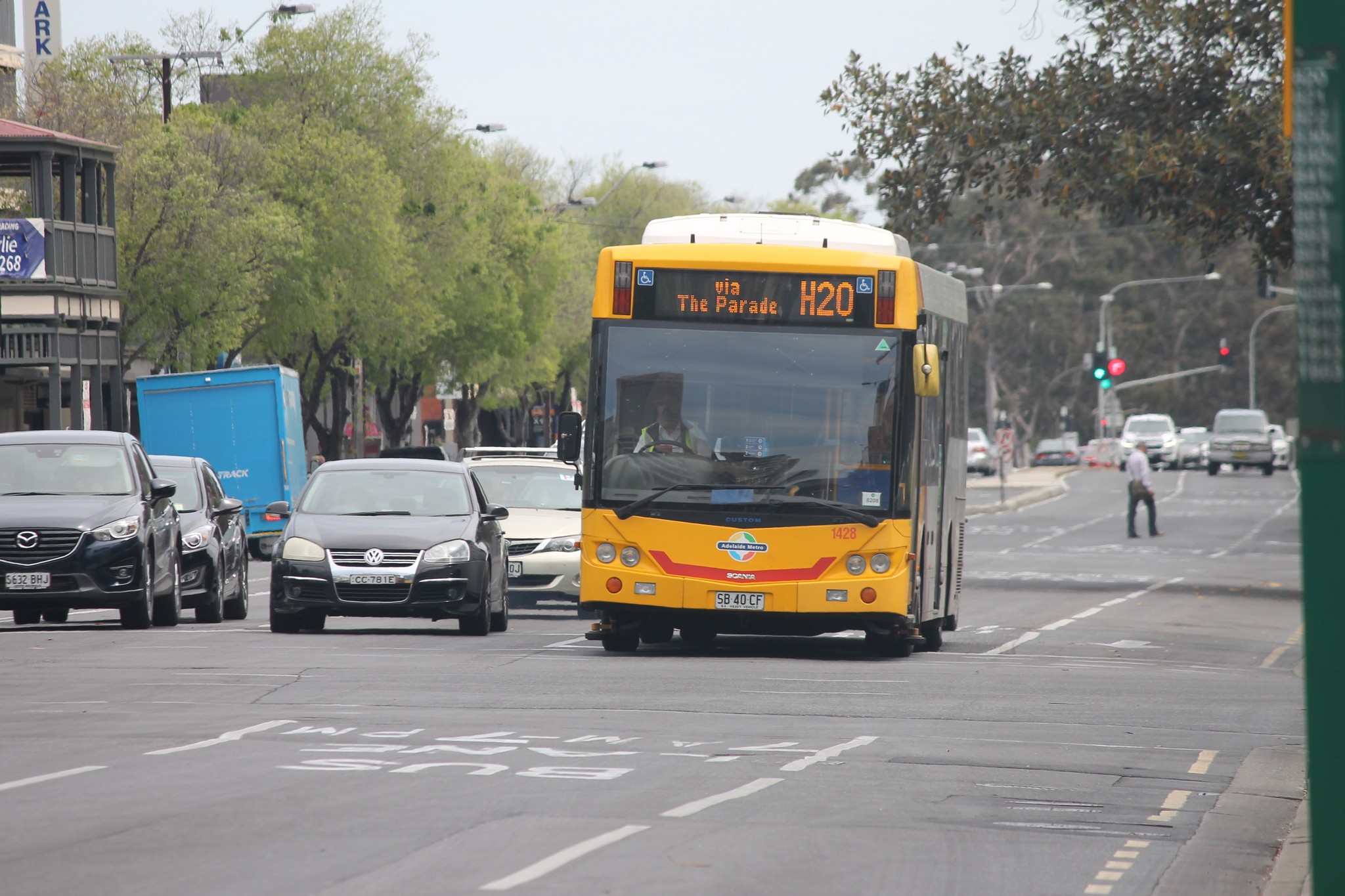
[1098,272,1231,439]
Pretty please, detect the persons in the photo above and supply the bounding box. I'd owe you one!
[633,396,712,459]
[1125,442,1164,538]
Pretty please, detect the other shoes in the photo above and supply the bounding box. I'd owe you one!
[1128,532,1165,538]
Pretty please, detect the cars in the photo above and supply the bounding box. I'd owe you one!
[455,446,601,620]
[1030,438,1078,468]
[966,426,998,476]
[265,457,510,635]
[146,454,249,625]
[1062,423,1296,470]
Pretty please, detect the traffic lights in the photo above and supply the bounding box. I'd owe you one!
[1094,350,1112,390]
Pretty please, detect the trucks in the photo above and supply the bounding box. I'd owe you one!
[138,365,320,563]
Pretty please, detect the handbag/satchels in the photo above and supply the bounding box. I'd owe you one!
[1132,481,1151,499]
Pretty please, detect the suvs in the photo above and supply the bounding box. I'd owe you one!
[0,430,185,630]
[1115,412,1183,472]
[1207,408,1276,476]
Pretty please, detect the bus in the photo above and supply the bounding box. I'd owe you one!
[557,213,969,660]
